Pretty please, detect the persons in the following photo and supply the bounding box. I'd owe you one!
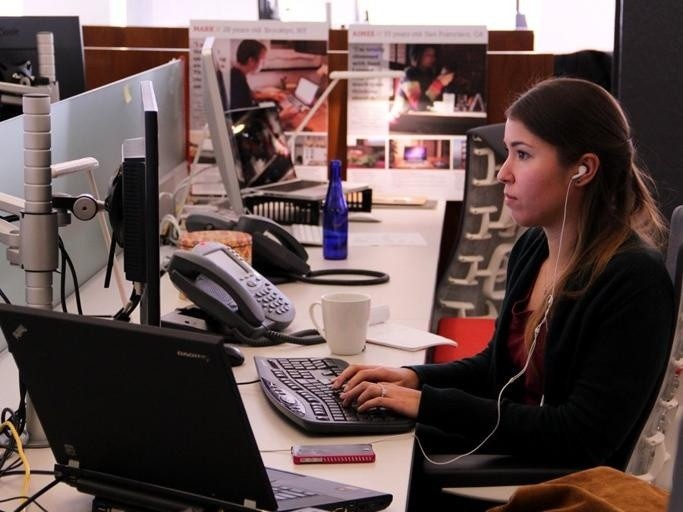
[388,45,456,133]
[231,37,304,124]
[327,76,676,511]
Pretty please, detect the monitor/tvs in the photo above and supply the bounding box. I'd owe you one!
[201,37,244,215]
[0,16,85,123]
[106,80,160,328]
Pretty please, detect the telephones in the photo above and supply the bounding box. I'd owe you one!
[168,205,310,337]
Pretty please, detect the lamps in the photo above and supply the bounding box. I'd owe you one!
[285,69,406,169]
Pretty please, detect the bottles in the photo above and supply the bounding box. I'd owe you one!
[322,159,348,260]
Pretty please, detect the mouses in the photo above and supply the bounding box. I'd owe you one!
[223,343,245,367]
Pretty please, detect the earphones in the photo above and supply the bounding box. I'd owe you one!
[572,165,587,180]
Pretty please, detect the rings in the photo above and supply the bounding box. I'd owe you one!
[376,382,385,397]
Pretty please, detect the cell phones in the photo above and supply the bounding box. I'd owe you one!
[292,444,375,464]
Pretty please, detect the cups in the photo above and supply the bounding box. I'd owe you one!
[310,292,372,355]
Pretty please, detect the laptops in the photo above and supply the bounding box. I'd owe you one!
[0,302,393,512]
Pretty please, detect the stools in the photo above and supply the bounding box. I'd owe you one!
[433,315,498,366]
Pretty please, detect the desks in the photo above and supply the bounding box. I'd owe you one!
[1,170,468,512]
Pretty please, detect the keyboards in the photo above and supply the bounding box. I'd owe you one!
[291,223,324,247]
[254,356,416,437]
